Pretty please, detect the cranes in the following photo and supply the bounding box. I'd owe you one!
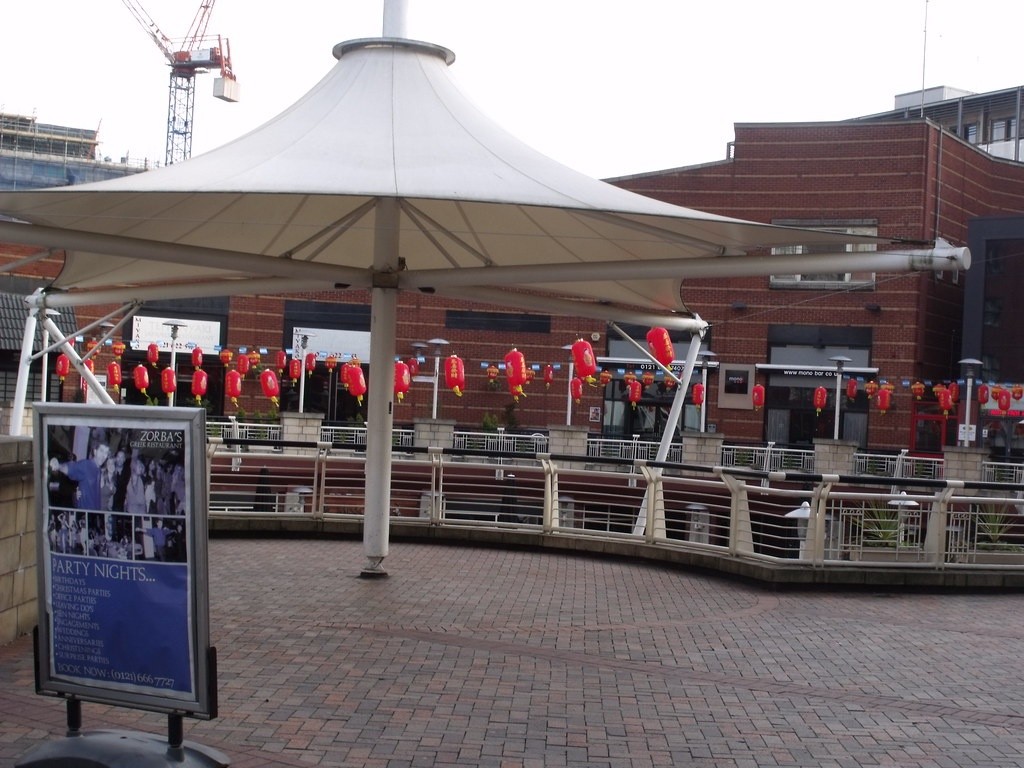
[122,0,240,104]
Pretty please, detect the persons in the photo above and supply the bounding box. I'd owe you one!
[51,444,186,516]
[136,520,184,563]
[49,510,86,557]
[88,527,132,561]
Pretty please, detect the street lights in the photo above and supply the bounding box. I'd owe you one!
[958,358,983,447]
[294,330,316,413]
[163,319,188,407]
[562,344,575,425]
[697,351,717,432]
[41,309,61,402]
[428,338,450,420]
[828,355,853,439]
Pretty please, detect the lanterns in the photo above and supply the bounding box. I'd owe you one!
[814,386,827,415]
[56,337,366,407]
[692,380,704,411]
[753,382,764,412]
[394,327,676,410]
[847,379,1022,419]
